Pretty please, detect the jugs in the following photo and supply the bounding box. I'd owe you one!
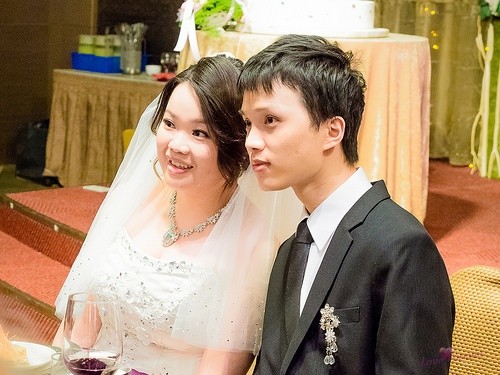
[120,23,141,74]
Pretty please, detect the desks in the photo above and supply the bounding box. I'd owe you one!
[176,25,431,230]
[44,69,169,188]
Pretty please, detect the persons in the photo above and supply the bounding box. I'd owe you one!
[50,56,303,375]
[236,33,456,375]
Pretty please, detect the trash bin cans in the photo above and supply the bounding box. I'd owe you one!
[14,118,56,187]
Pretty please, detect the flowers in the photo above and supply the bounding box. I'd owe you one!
[174,1,253,68]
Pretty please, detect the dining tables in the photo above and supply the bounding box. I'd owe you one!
[0,341,150,375]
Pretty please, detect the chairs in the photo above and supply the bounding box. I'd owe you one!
[447,266,500,375]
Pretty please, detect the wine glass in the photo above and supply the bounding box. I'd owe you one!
[161,53,176,73]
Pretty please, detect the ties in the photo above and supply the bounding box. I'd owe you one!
[284,221,314,353]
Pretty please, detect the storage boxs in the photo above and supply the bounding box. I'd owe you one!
[70,51,147,74]
[116,22,149,74]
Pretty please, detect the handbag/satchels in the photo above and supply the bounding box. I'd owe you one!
[14,120,61,186]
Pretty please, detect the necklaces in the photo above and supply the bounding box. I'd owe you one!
[162,188,226,247]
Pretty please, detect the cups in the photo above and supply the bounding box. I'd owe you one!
[145,65,161,75]
